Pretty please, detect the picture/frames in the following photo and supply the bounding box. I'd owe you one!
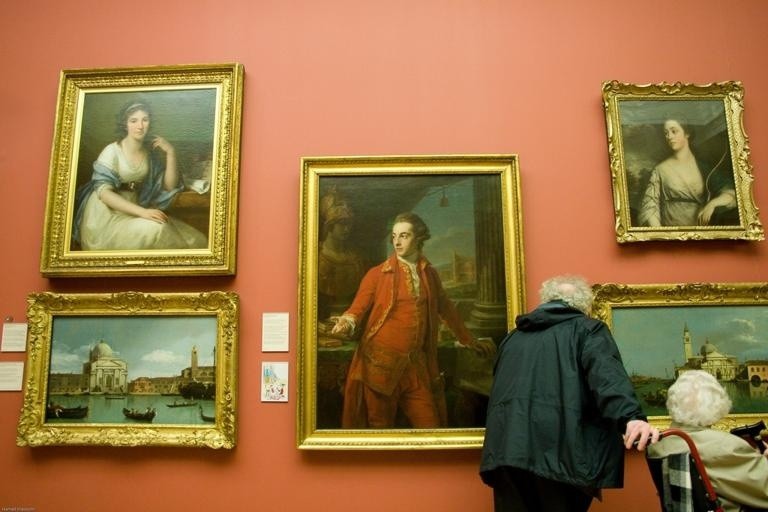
[297,153,526,454]
[14,290,238,449]
[41,62,245,278]
[602,77,765,244]
[591,280,768,439]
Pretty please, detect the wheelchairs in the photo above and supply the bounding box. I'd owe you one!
[630,427,723,511]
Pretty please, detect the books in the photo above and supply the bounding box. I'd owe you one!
[318,336,343,347]
[318,319,335,331]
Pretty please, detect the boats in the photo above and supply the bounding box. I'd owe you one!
[166,399,198,407]
[160,392,180,396]
[200,406,214,421]
[106,396,125,400]
[47,401,89,419]
[122,406,157,422]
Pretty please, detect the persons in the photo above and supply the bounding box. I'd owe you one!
[635,111,738,226]
[72,98,207,249]
[329,213,496,428]
[664,369,768,511]
[319,191,365,295]
[480,273,660,511]
